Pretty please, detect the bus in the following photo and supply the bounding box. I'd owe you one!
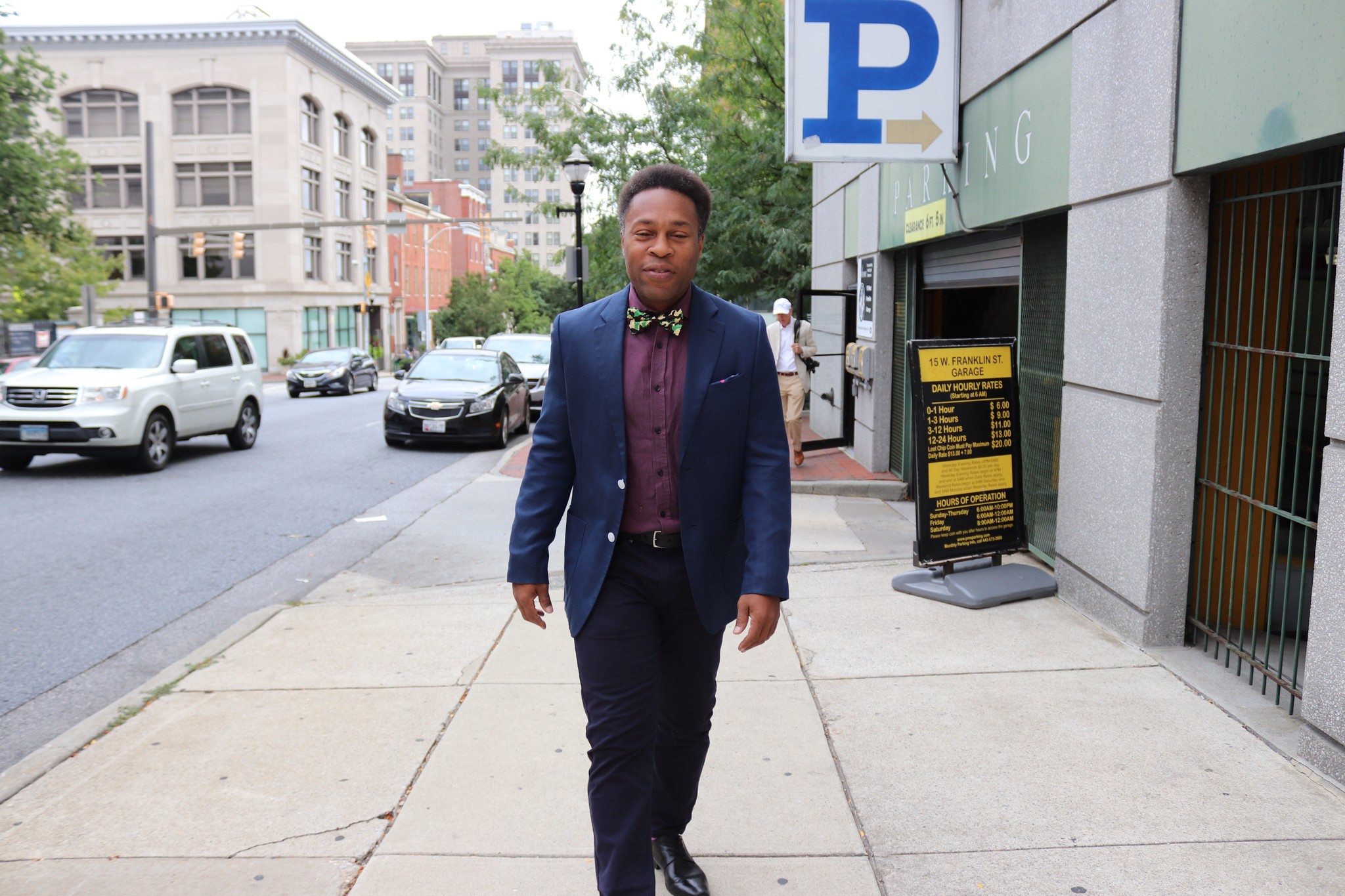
[286,347,379,398]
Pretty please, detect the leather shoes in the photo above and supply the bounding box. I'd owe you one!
[652,835,710,896]
[793,446,805,466]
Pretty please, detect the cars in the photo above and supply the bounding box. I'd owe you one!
[383,349,531,450]
[439,336,486,349]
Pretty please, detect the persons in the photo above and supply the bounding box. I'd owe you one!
[508,162,792,896]
[765,297,817,466]
[405,346,414,360]
[411,347,421,359]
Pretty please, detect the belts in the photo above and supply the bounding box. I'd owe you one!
[777,371,798,377]
[616,530,682,548]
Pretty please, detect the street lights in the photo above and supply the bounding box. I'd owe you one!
[543,88,626,190]
[563,143,593,308]
[424,224,465,352]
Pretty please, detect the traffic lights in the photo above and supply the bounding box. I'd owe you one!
[229,232,246,260]
[363,218,378,251]
[189,232,207,259]
[353,303,371,314]
[155,293,175,311]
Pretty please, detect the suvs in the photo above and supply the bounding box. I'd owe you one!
[482,333,552,422]
[0,318,263,472]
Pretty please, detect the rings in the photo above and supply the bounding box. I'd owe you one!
[793,349,795,351]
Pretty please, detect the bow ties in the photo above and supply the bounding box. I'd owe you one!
[627,307,684,337]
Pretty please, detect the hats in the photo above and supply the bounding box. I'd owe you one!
[773,298,792,315]
